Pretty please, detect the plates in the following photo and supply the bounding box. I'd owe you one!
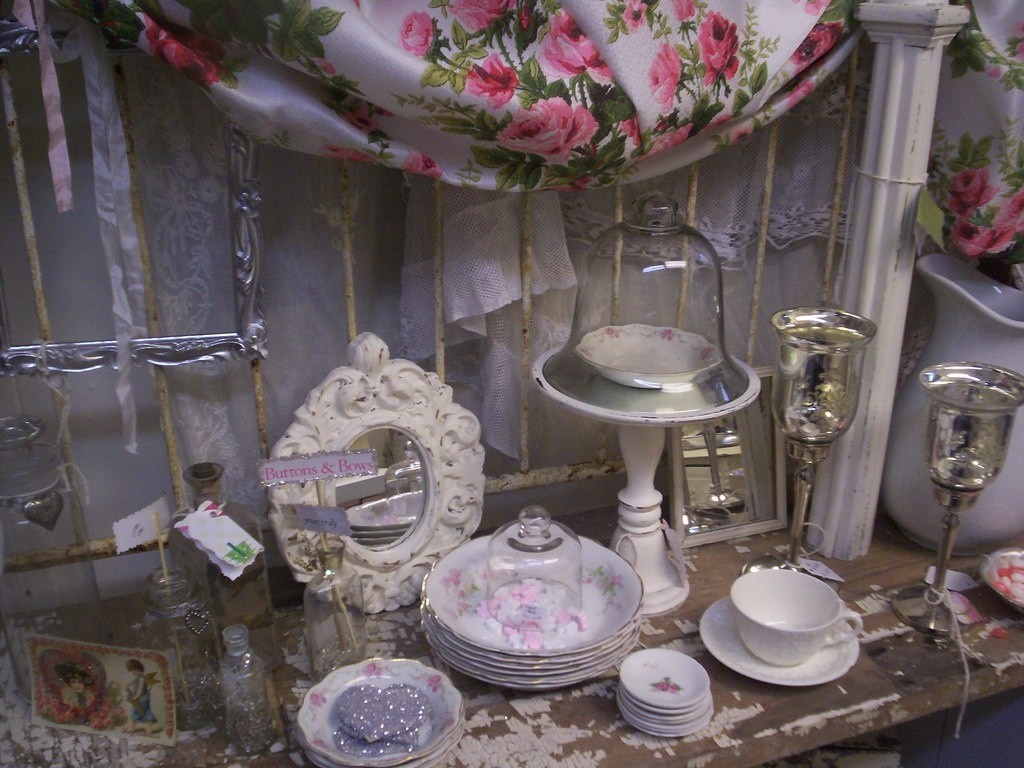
[297,658,466,768]
[979,548,1024,613]
[615,648,713,737]
[699,595,860,686]
[420,535,643,690]
[574,323,724,389]
[346,487,423,545]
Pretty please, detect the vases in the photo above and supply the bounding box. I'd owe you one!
[881,251,1024,558]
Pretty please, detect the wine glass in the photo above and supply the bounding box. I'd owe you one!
[892,361,1024,637]
[681,457,732,537]
[695,423,744,509]
[742,306,879,594]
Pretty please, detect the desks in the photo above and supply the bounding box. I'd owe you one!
[0,507,1024,768]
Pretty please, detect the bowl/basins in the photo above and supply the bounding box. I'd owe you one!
[719,430,741,446]
[728,468,746,490]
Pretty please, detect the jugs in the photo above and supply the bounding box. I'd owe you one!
[879,252,1024,556]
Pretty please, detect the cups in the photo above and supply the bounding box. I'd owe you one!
[730,569,863,666]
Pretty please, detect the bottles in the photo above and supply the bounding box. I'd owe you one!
[0,412,113,706]
[168,463,283,673]
[219,624,276,756]
[303,537,367,686]
[138,564,221,733]
